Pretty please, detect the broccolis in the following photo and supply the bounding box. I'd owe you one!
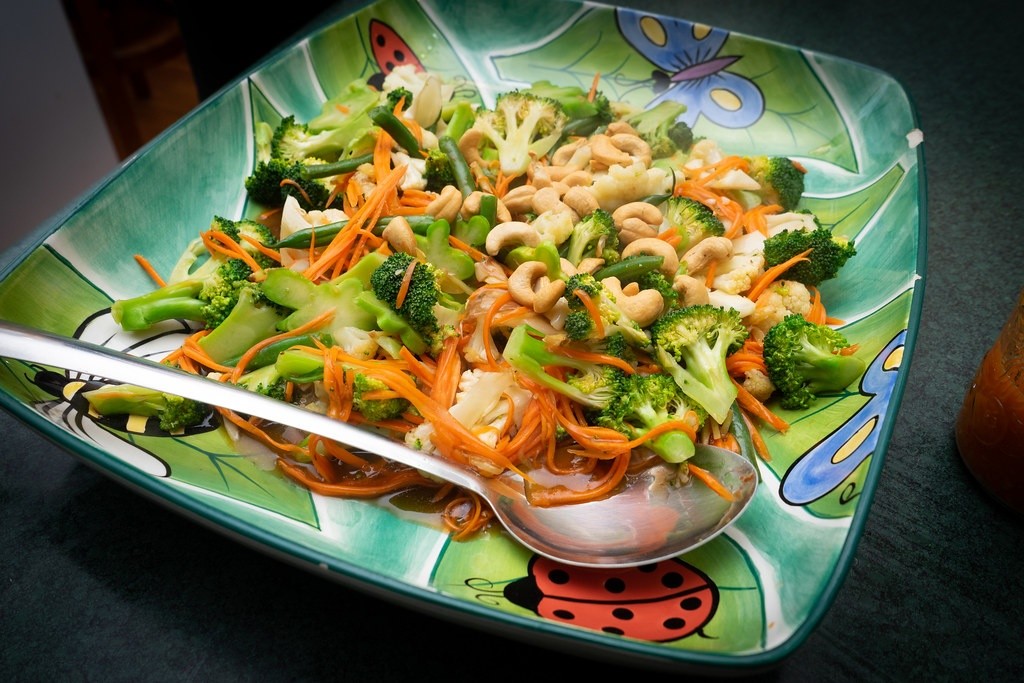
[82,76,867,466]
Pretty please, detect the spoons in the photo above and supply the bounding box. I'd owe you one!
[1,319,757,568]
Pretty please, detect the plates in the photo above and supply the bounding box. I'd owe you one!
[0,0,928,666]
